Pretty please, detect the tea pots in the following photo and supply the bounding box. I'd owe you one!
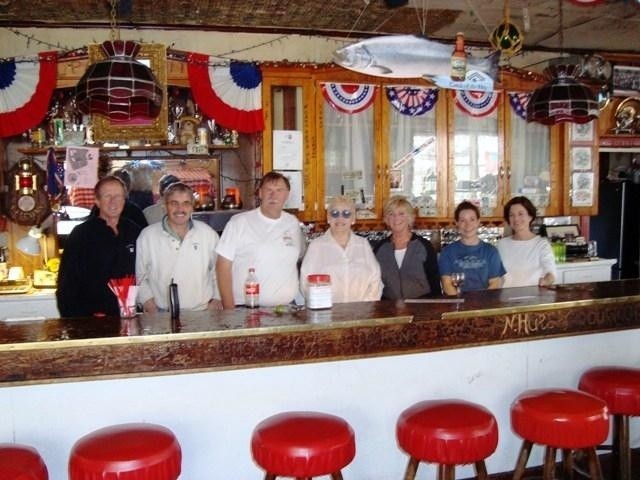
[192,184,214,212]
[222,188,245,209]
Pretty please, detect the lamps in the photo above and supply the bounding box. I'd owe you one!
[526,0,600,125]
[15,223,49,264]
[76,0,163,121]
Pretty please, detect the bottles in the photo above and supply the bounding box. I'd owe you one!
[246,268,261,310]
[304,274,334,311]
[170,285,180,320]
[450,31,467,81]
[21,89,86,144]
[1,248,8,280]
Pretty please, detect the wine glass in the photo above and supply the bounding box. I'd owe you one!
[452,272,465,299]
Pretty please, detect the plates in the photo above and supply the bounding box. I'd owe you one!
[614,96,639,131]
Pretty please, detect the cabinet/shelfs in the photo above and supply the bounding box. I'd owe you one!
[262,75,317,223]
[563,267,612,284]
[449,88,563,223]
[315,80,448,231]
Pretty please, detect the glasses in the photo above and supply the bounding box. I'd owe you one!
[329,209,353,219]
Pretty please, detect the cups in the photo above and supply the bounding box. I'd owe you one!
[9,267,24,279]
[119,305,137,319]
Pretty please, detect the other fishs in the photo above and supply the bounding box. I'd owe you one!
[332,34,502,84]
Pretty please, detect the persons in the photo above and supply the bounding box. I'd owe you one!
[496,196,556,288]
[300,198,383,303]
[374,198,442,302]
[438,202,506,296]
[56,154,224,319]
[215,173,308,311]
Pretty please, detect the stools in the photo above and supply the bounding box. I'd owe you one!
[1,442,49,480]
[511,388,610,479]
[577,365,640,479]
[393,398,499,480]
[249,411,356,480]
[68,423,183,480]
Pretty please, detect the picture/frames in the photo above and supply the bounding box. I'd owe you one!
[569,115,594,208]
